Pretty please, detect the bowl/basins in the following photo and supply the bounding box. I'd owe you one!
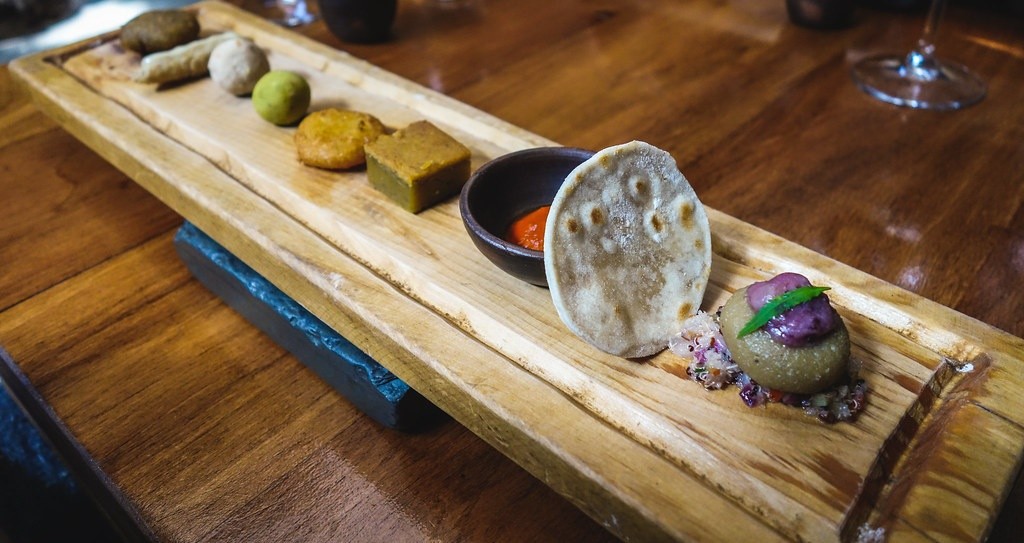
[459,146,601,288]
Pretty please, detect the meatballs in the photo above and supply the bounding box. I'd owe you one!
[253,70,311,124]
[207,39,270,95]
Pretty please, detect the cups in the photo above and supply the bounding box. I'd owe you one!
[318,2,399,43]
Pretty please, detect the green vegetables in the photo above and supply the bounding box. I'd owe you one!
[738,286,830,337]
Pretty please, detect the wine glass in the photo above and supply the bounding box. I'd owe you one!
[852,0,986,108]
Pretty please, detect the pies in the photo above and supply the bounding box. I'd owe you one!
[544,140,712,361]
